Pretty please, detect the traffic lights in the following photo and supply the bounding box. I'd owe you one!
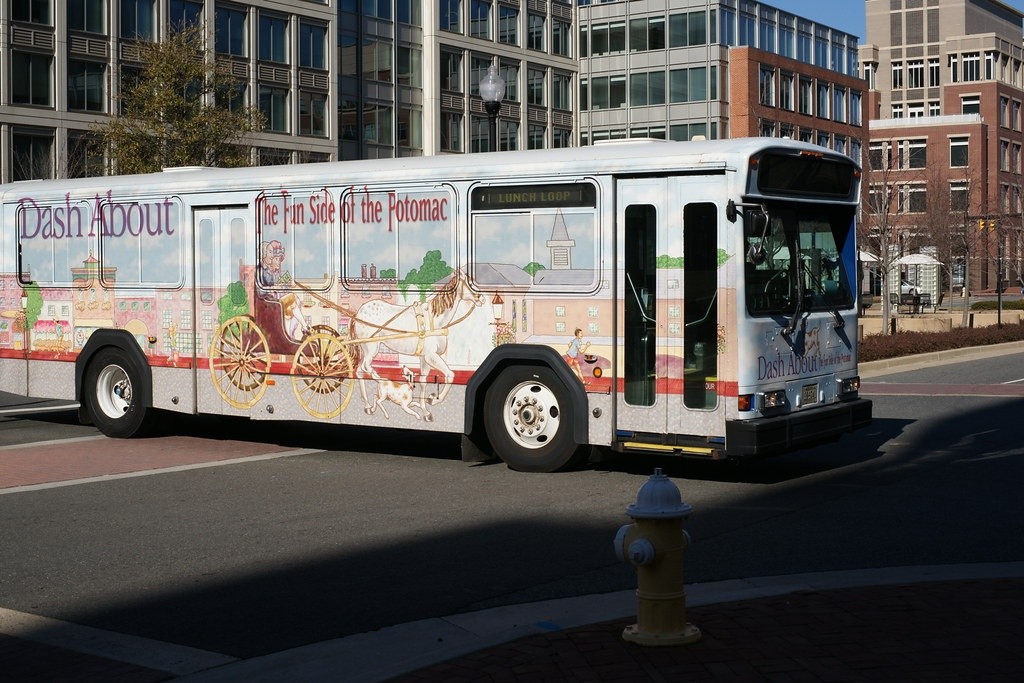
[989,220,995,232]
[979,219,985,230]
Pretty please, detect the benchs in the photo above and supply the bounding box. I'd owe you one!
[861,294,873,315]
[900,293,931,314]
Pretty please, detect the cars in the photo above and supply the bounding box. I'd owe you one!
[901,280,920,295]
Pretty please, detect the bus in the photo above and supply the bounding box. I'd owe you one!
[0,133,871,474]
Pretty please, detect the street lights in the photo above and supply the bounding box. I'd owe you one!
[480,65,506,152]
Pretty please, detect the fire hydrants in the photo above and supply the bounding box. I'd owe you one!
[615,466,703,646]
[962,287,966,297]
